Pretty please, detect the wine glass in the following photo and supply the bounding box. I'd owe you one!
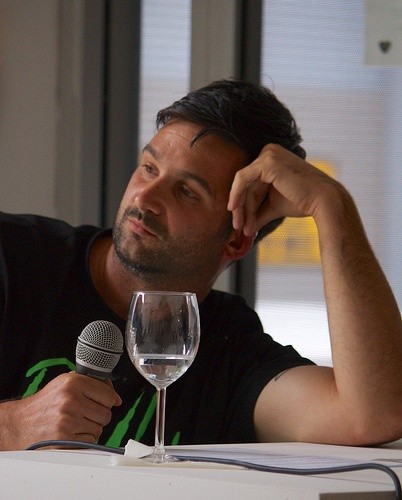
[124,290,201,463]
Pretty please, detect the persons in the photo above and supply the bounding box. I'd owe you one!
[0,76,402,451]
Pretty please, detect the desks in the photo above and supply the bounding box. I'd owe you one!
[1,440,402,500]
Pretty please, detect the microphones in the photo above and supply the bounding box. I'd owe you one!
[76,320,125,381]
